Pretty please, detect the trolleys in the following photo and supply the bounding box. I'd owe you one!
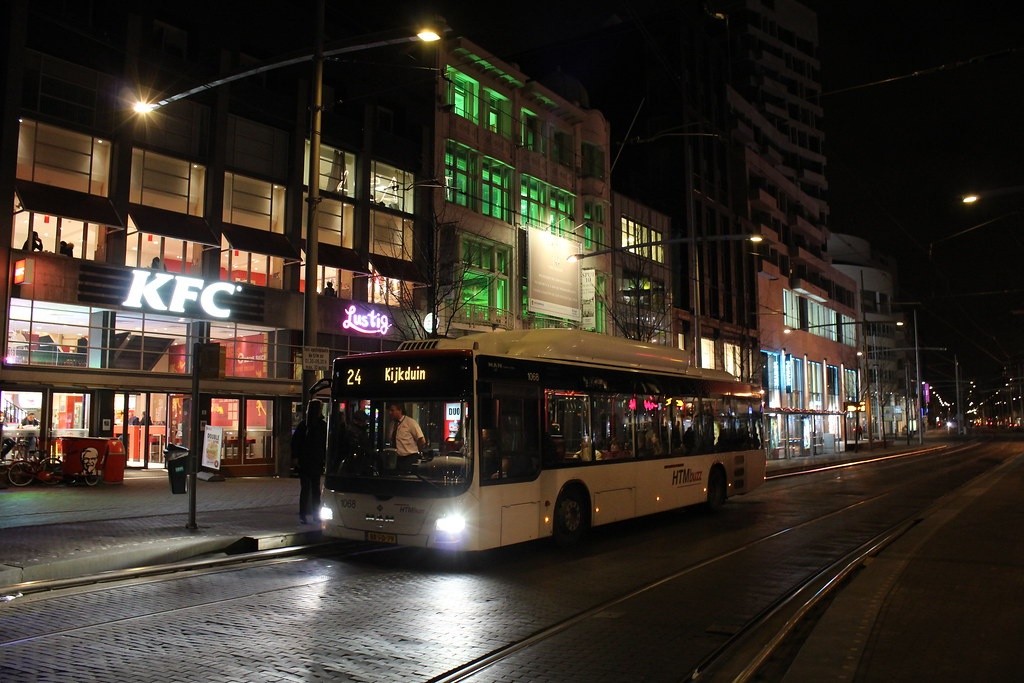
[61,436,111,487]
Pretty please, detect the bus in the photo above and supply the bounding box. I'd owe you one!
[303,328,770,558]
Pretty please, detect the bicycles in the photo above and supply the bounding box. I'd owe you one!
[7,440,64,488]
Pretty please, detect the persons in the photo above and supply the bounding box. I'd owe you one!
[128,412,141,426]
[480,420,761,484]
[320,281,337,297]
[0,410,16,461]
[140,410,152,426]
[151,257,167,271]
[18,413,40,453]
[21,230,43,251]
[386,399,429,475]
[327,408,372,478]
[59,241,74,257]
[289,399,328,524]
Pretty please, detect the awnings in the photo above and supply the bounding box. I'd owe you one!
[9,178,434,292]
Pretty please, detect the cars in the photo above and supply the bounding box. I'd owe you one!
[773,438,810,457]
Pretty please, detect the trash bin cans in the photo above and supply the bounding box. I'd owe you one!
[163,448,191,495]
[100,437,124,484]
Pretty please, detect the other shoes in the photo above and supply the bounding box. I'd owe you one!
[298,516,306,525]
[313,514,320,521]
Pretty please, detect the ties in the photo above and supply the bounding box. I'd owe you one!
[390,419,397,448]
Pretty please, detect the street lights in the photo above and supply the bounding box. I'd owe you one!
[567,234,765,368]
[783,320,904,452]
[855,347,952,444]
[130,28,444,524]
[910,366,1024,437]
